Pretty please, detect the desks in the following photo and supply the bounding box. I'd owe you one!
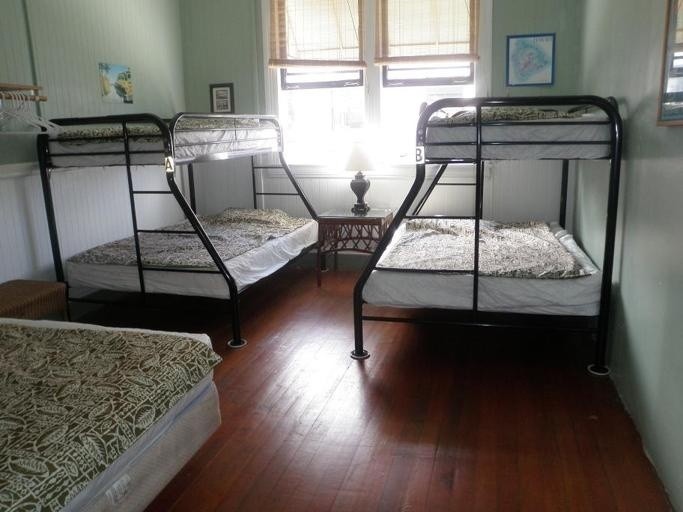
[317,208,393,287]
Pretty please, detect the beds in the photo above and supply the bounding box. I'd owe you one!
[350,95,623,377]
[0,317,222,512]
[36,111,328,349]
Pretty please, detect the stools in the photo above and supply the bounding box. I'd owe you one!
[0,280,68,320]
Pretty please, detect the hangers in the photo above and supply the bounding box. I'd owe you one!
[0,90,63,135]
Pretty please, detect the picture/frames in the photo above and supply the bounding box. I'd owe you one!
[209,82,234,114]
[654,0,683,126]
[505,32,557,87]
